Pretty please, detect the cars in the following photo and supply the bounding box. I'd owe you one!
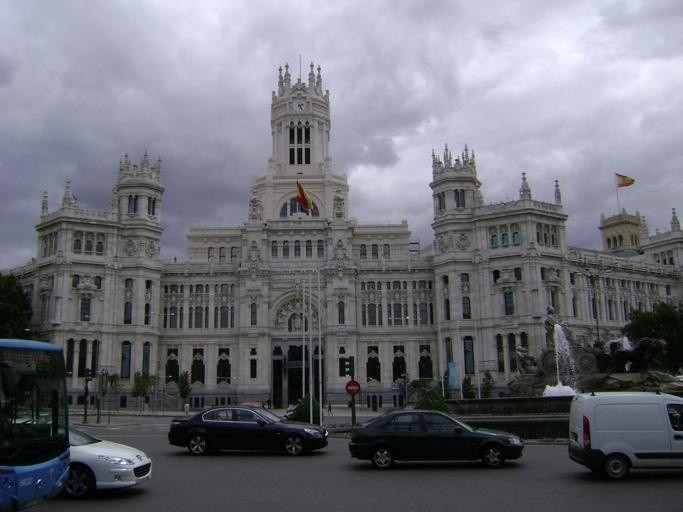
[347,409,525,469]
[9,412,155,498]
[167,406,330,456]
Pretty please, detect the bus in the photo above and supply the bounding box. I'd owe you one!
[0,337,73,511]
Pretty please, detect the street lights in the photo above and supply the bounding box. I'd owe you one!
[574,265,613,341]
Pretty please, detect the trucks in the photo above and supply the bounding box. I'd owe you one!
[568,387,683,480]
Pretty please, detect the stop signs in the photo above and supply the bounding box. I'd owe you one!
[346,381,360,394]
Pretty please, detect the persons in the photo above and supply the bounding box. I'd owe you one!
[326,401,333,417]
[266,397,272,410]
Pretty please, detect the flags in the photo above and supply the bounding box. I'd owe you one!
[295,181,313,210]
[615,173,636,187]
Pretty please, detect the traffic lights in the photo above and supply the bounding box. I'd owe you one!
[85,368,94,382]
[343,355,355,376]
